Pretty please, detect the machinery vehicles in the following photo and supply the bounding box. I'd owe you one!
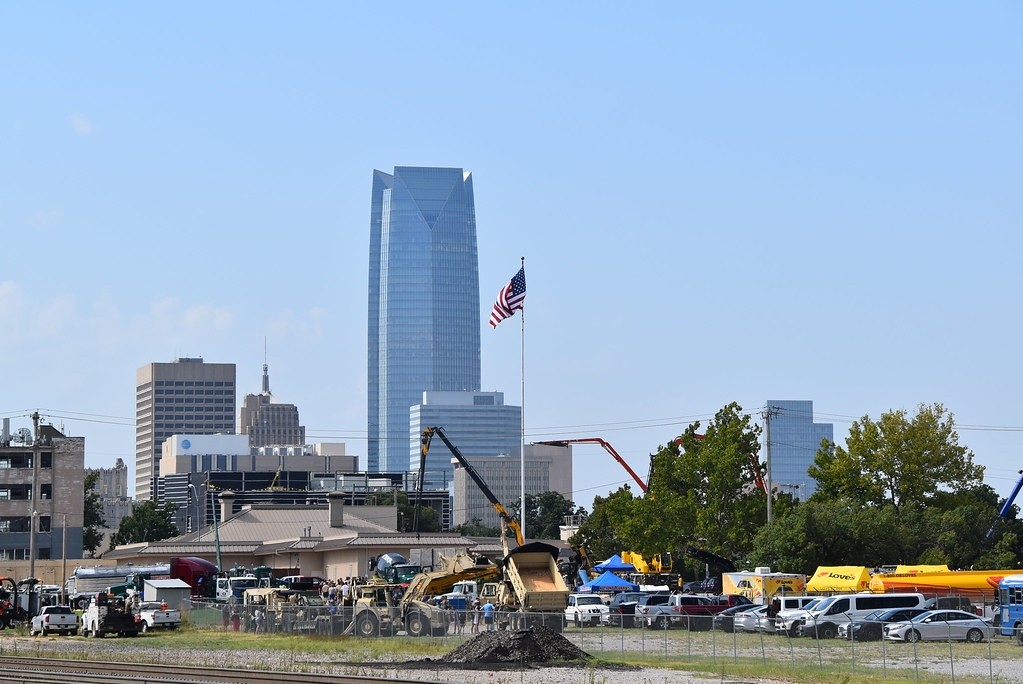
[211,423,570,641]
[613,549,682,595]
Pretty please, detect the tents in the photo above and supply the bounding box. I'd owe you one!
[577,571,639,603]
[594,553,636,584]
[895,564,949,574]
[806,566,872,598]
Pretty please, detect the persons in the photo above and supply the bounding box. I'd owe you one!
[614,571,631,583]
[391,585,403,606]
[459,612,467,635]
[470,597,482,634]
[319,576,367,614]
[222,605,230,631]
[242,606,297,635]
[452,605,460,634]
[427,594,450,620]
[480,600,495,632]
[232,605,240,631]
[677,573,684,593]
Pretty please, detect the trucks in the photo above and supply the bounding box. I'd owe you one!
[67,555,223,609]
[722,565,807,607]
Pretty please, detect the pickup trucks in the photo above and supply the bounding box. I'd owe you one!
[130,600,183,634]
[28,604,80,638]
[80,592,139,639]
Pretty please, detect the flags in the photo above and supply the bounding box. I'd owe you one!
[488,268,526,329]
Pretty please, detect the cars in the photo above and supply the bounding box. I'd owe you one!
[565,567,997,644]
[279,575,328,597]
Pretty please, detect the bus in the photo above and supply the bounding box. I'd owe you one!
[998,573,1023,647]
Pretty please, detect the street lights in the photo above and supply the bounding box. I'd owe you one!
[187,483,206,542]
